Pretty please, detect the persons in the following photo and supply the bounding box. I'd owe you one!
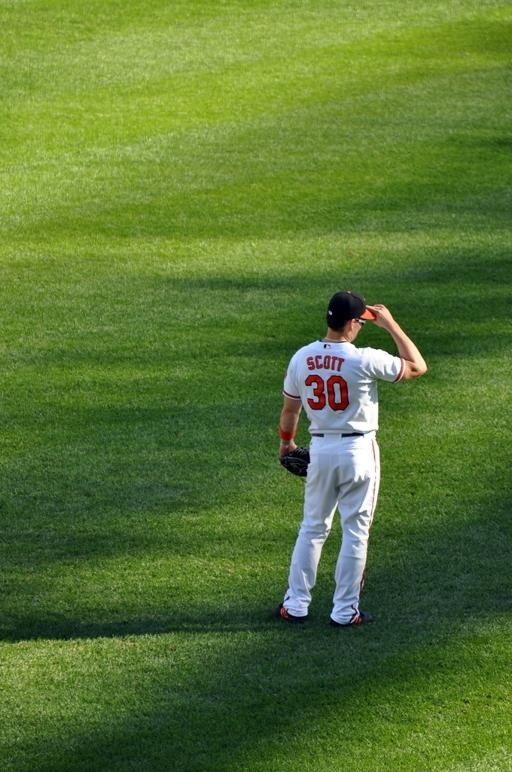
[276,290,426,626]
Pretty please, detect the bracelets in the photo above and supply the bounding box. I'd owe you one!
[278,426,296,440]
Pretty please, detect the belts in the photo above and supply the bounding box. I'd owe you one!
[312,433,364,437]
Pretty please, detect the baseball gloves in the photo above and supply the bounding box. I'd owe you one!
[281,447,309,476]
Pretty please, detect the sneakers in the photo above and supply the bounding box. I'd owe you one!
[278,602,309,622]
[329,613,365,627]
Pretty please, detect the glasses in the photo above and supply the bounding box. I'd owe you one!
[355,318,367,326]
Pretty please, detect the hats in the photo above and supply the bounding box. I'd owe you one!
[327,291,376,320]
